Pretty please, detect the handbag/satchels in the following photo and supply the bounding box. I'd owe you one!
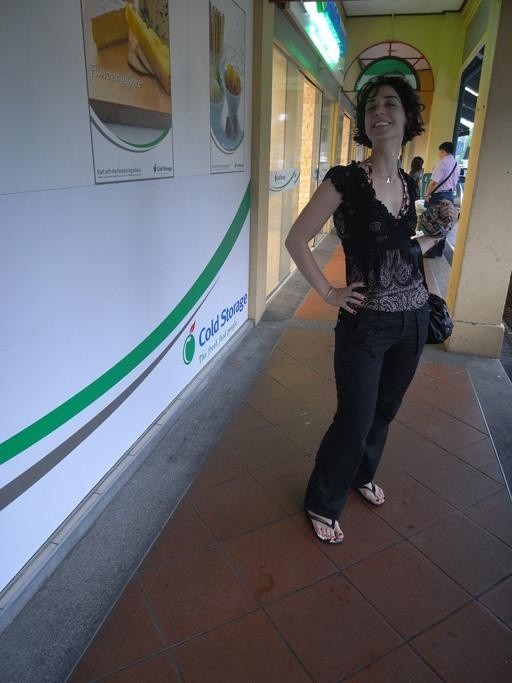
[427,291,455,344]
[419,205,454,237]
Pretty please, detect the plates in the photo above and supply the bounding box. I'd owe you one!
[212,127,244,154]
[89,107,173,152]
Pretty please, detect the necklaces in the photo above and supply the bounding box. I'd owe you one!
[378,173,397,185]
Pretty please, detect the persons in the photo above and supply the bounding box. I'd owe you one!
[282,72,460,544]
[421,142,461,258]
[408,155,425,198]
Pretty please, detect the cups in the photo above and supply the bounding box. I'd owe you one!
[210,90,241,140]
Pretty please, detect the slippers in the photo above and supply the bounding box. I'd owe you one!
[303,508,344,546]
[358,480,387,507]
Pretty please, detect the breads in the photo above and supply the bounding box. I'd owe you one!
[124,4,171,94]
[91,8,129,50]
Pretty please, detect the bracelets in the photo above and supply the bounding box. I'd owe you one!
[325,286,334,303]
